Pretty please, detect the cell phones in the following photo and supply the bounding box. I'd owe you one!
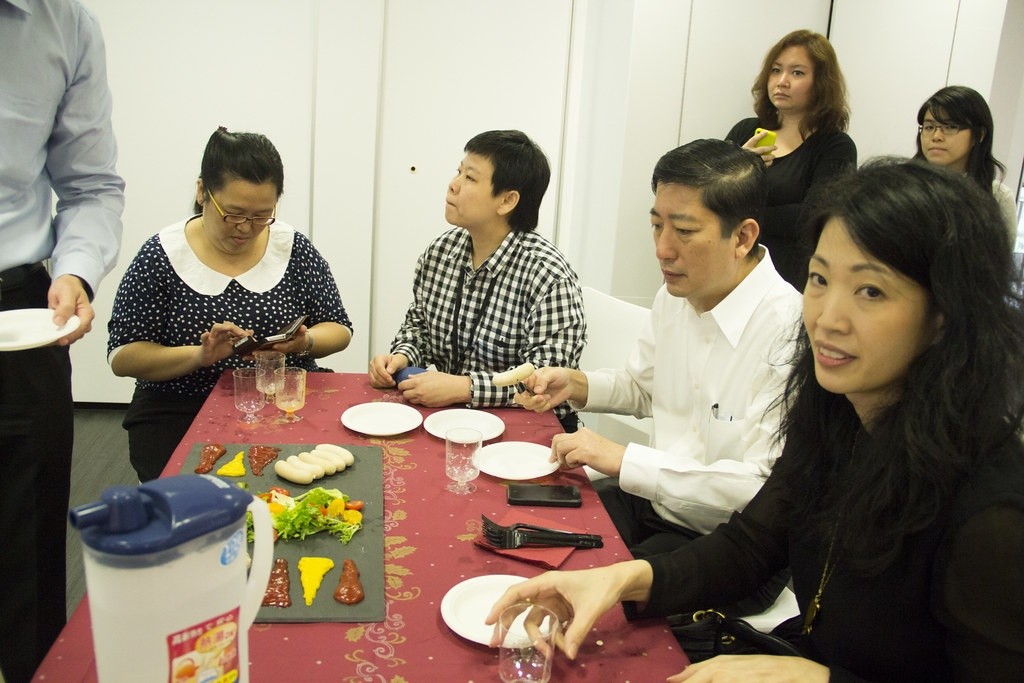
[754,127,776,156]
[507,484,582,507]
[263,333,287,342]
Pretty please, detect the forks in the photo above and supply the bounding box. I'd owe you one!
[481,513,604,549]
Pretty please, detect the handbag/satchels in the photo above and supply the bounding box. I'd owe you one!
[665,609,804,662]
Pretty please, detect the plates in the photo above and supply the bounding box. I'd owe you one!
[341,402,423,438]
[423,408,505,444]
[1,308,80,351]
[440,574,567,649]
[472,441,561,480]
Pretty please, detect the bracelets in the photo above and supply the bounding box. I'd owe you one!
[295,332,313,357]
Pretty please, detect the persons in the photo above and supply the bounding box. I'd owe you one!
[368,130,590,434]
[484,155,1024,682]
[0,1,125,683]
[909,85,1017,251]
[105,125,354,484]
[512,138,808,563]
[722,29,858,293]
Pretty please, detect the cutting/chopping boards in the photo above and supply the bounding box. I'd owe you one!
[176,441,386,623]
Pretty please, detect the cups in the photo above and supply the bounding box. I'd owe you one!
[499,604,559,683]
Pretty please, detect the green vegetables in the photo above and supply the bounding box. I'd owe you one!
[246,488,363,544]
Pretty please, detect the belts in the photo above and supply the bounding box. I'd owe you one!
[1,261,44,292]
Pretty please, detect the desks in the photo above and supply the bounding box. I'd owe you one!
[31,371,690,683]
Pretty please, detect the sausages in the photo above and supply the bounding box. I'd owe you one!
[492,362,534,388]
[275,443,355,485]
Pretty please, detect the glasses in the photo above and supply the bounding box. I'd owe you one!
[917,120,972,136]
[206,186,276,226]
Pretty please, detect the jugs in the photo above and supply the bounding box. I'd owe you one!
[69,473,274,683]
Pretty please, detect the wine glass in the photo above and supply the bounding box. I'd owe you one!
[255,350,286,406]
[233,367,266,424]
[273,366,307,423]
[445,427,482,494]
